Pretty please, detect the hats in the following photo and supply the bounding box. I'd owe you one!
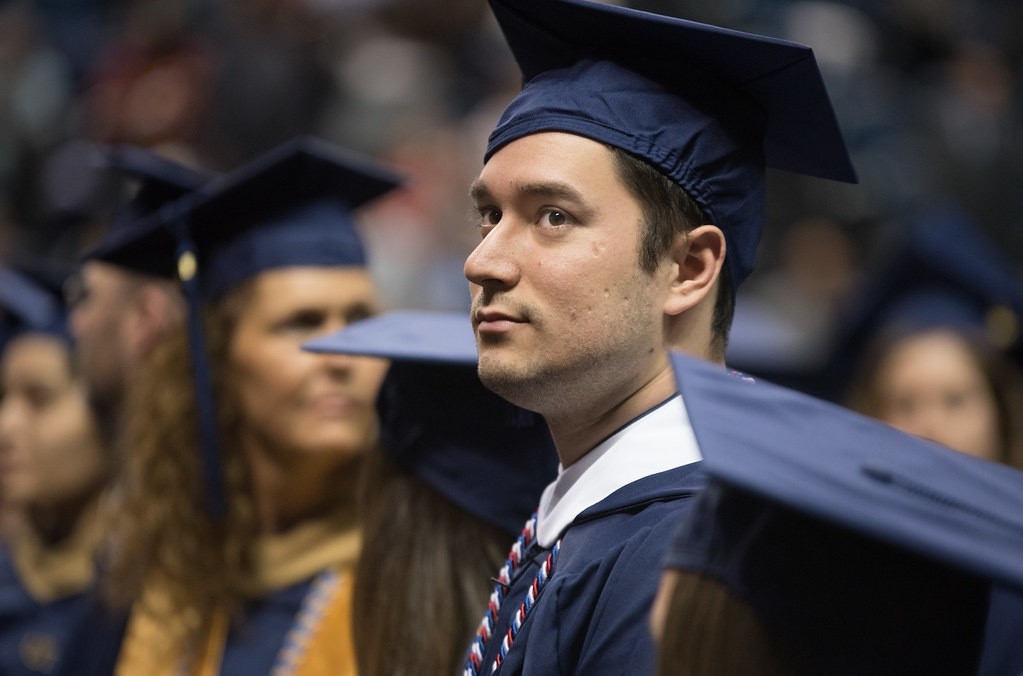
[88,142,207,238]
[79,140,406,517]
[0,264,78,355]
[301,313,560,540]
[484,0,859,289]
[661,351,1023,676]
[843,213,1001,367]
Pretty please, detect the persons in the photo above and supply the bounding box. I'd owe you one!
[0,1,1023,674]
